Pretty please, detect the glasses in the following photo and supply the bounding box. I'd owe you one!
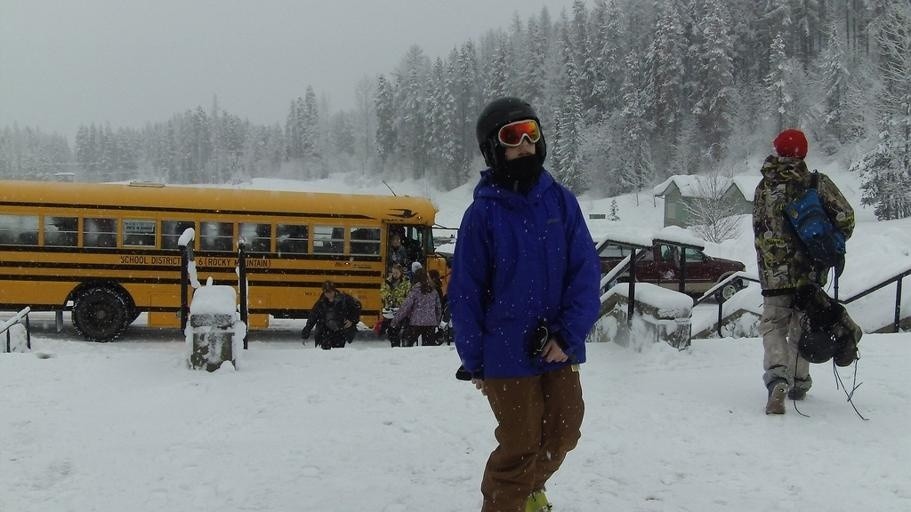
[495,119,542,148]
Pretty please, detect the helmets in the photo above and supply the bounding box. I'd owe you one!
[799,329,835,363]
[476,97,546,169]
[322,280,336,292]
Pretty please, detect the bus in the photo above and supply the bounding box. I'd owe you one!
[0,178,452,343]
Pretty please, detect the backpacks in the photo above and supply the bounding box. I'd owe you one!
[342,293,361,325]
[785,169,847,273]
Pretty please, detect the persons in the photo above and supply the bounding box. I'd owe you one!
[301,280,361,350]
[255,222,380,261]
[376,226,442,347]
[445,96,604,512]
[750,128,856,416]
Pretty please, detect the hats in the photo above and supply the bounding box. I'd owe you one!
[774,130,808,159]
[411,262,422,273]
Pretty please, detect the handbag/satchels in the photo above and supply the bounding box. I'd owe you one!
[383,307,400,319]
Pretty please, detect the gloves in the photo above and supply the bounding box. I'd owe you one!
[438,322,447,331]
[301,327,311,339]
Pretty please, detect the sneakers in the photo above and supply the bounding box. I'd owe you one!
[525,490,550,512]
[788,382,808,401]
[765,378,789,415]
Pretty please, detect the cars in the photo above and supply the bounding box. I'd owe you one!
[592,228,749,304]
